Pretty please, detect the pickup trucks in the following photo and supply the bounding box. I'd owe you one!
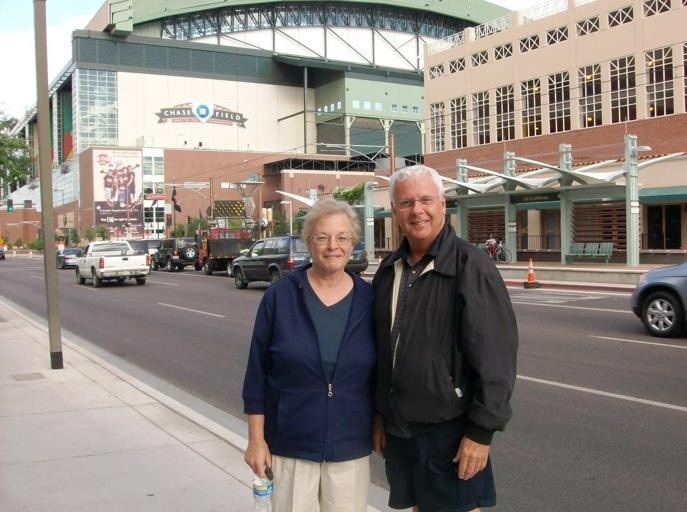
[76,241,151,287]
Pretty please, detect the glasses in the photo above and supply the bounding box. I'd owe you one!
[312,235,354,245]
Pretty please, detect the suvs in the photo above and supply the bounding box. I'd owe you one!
[231,235,369,289]
[143,238,202,272]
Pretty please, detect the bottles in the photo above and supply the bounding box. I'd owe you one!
[252,466,273,512]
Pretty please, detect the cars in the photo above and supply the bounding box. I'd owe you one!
[57,248,83,270]
[631,259,687,337]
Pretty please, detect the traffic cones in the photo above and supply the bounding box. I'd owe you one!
[523,259,539,288]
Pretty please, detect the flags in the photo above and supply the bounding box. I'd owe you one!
[172,190,182,212]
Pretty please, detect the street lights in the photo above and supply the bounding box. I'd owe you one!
[171,186,177,230]
[280,200,292,253]
[54,189,66,246]
[624,133,652,266]
[230,181,264,233]
[146,199,156,237]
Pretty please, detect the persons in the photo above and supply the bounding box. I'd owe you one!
[242,197,376,511]
[370,164,518,512]
[58,240,64,249]
[104,164,136,205]
[486,232,497,256]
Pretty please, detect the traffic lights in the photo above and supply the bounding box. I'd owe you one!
[188,215,192,223]
[6,199,13,211]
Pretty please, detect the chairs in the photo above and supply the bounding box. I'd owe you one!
[566,242,614,263]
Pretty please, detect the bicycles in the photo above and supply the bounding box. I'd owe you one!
[476,236,512,263]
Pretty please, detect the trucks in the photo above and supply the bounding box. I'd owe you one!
[198,227,255,278]
